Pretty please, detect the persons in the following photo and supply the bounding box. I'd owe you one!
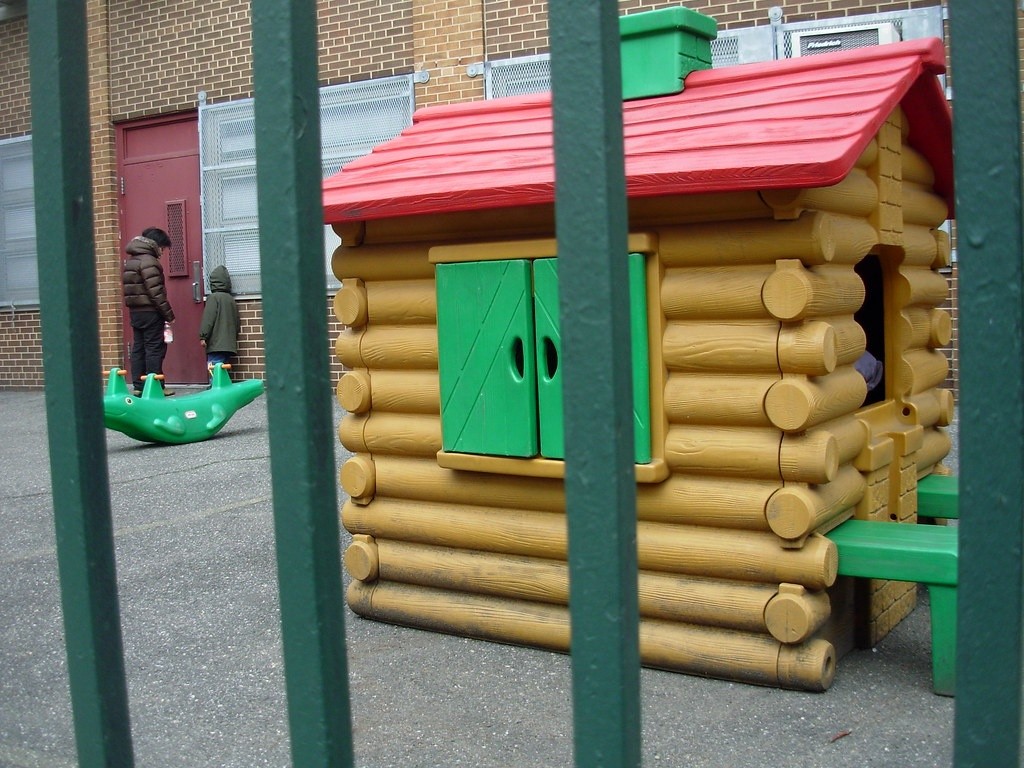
[199,265,239,390]
[123,227,176,398]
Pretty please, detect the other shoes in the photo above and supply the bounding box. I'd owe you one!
[206,385,212,390]
[163,388,175,396]
[134,390,142,398]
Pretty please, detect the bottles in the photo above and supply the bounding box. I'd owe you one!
[163,321,174,343]
